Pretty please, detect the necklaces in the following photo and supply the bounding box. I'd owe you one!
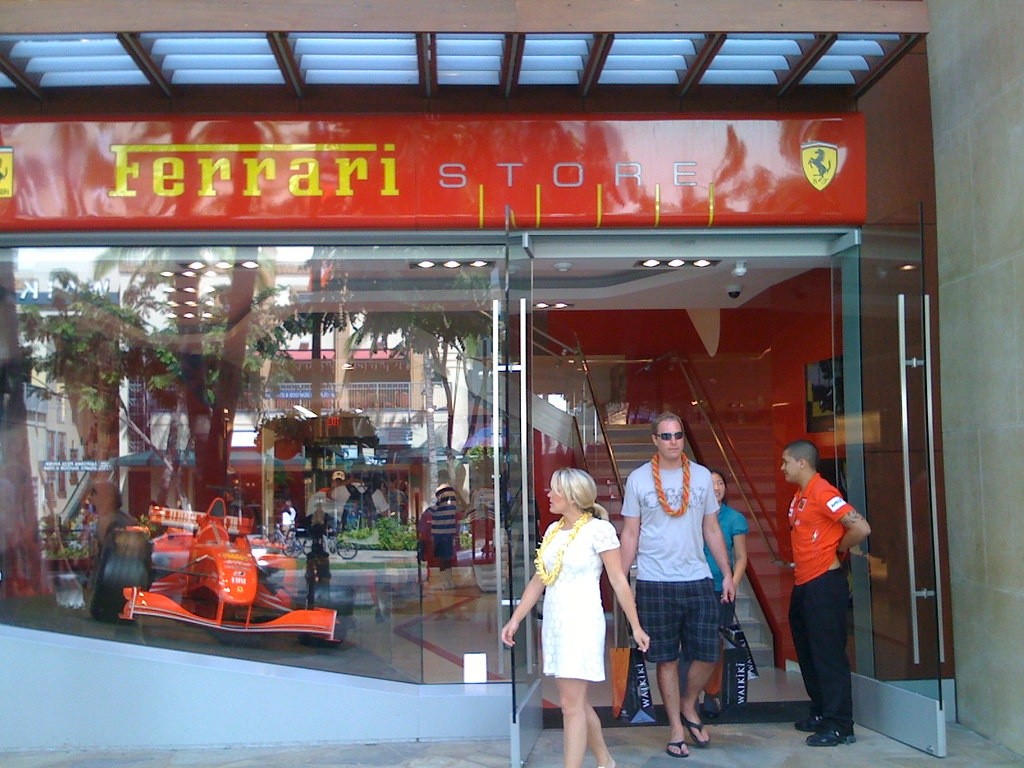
[534,512,587,584]
[651,451,690,516]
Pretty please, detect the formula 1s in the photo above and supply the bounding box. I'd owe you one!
[88,496,343,650]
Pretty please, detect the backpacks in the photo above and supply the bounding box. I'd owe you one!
[341,483,378,539]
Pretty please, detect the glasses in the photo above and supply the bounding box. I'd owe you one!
[656,432,684,441]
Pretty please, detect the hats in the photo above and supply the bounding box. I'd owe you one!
[331,471,345,480]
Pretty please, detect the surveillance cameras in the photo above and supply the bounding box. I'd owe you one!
[728,285,742,299]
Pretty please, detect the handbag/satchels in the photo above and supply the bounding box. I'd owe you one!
[723,598,759,682]
[609,609,657,726]
[704,600,748,714]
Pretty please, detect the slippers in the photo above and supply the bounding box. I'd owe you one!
[667,741,689,758]
[679,712,711,746]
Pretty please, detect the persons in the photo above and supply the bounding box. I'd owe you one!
[431,470,461,567]
[501,468,650,768]
[306,463,424,628]
[282,504,291,536]
[206,487,247,517]
[620,411,736,757]
[781,440,871,746]
[93,482,136,538]
[0,441,35,599]
[702,470,750,718]
[417,495,471,621]
[286,501,296,529]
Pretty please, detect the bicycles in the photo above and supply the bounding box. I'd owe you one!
[255,523,357,560]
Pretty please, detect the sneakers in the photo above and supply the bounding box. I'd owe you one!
[795,714,824,732]
[806,727,856,747]
